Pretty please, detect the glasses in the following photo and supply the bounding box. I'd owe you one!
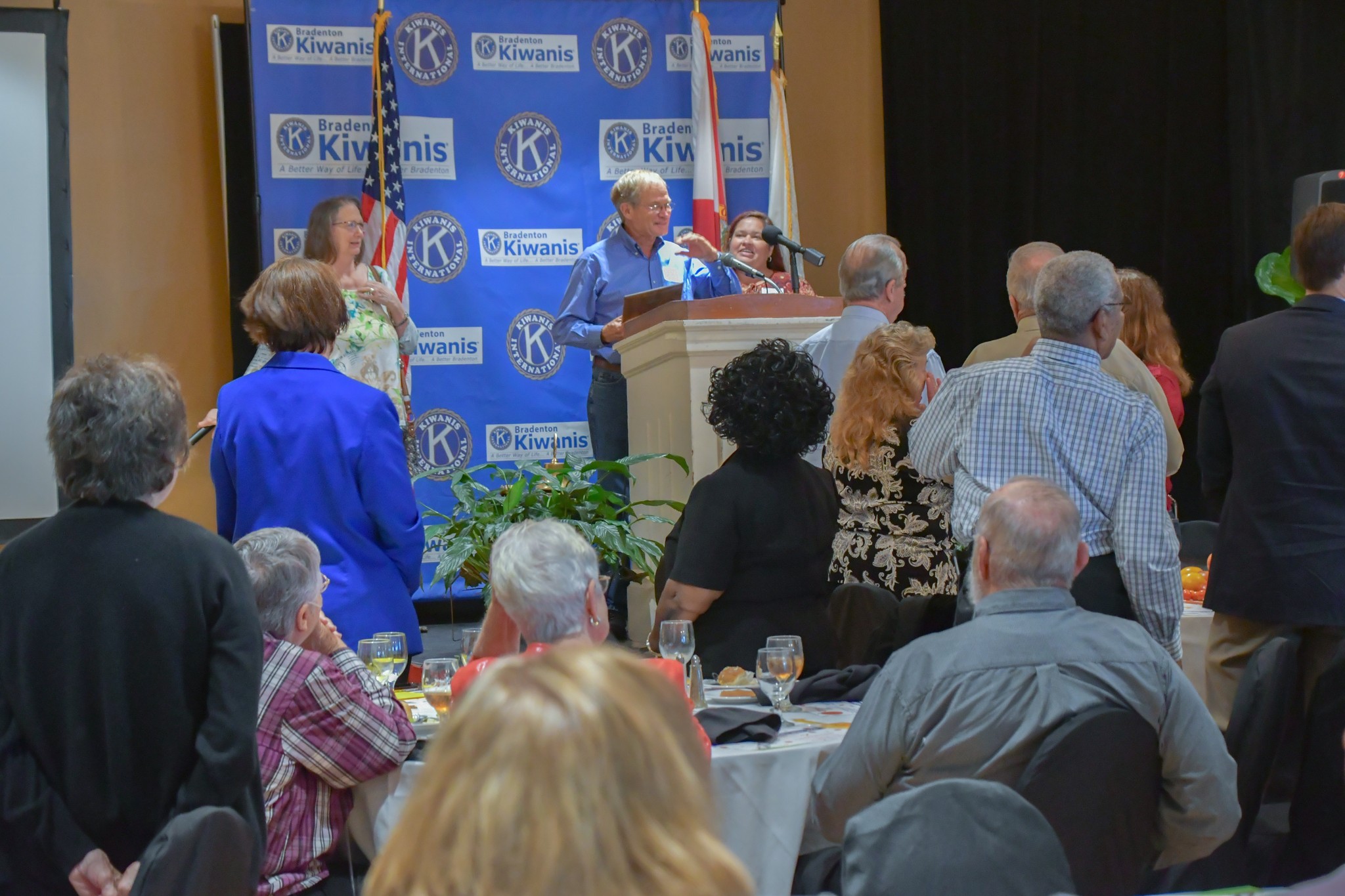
[317,574,329,593]
[632,200,675,215]
[1091,296,1133,322]
[333,221,367,232]
[599,573,611,592]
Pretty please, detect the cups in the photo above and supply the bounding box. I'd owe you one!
[460,628,482,667]
[421,658,460,714]
[357,637,394,685]
[659,620,695,663]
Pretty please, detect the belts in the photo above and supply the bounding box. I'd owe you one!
[593,357,621,373]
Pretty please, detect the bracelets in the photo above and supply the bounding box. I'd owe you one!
[715,252,721,261]
[647,632,663,656]
[391,310,408,327]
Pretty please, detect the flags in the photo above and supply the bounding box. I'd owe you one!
[353,20,412,418]
[764,69,807,282]
[688,11,727,260]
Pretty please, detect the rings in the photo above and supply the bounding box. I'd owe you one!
[370,287,374,294]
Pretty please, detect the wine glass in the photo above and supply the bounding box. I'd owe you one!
[755,647,798,727]
[766,635,804,714]
[373,633,408,691]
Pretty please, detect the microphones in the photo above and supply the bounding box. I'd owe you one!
[721,251,765,278]
[761,223,824,268]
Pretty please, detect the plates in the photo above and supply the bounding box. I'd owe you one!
[703,678,758,689]
[412,716,440,731]
[704,689,757,703]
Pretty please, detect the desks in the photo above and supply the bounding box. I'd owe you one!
[333,651,873,895]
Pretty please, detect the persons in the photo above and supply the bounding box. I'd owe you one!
[206,259,424,687]
[817,321,960,598]
[1192,203,1345,727]
[791,234,950,464]
[647,336,842,683]
[790,476,1241,895]
[724,211,815,297]
[196,198,418,434]
[1105,268,1192,556]
[231,527,417,895]
[963,242,1186,479]
[0,349,270,896]
[552,171,742,633]
[453,520,712,767]
[358,643,759,896]
[906,250,1183,672]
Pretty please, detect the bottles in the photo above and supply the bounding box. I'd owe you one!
[689,653,709,707]
